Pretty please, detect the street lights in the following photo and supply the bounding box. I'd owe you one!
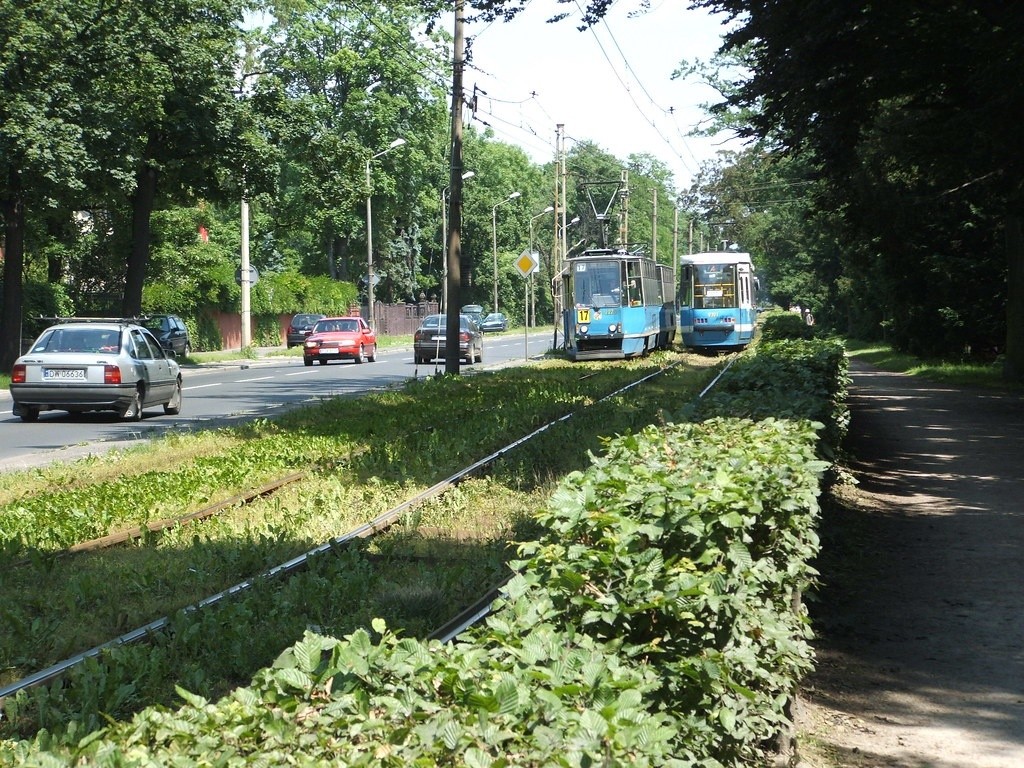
[492,191,521,313]
[529,206,554,329]
[442,170,476,317]
[365,138,407,332]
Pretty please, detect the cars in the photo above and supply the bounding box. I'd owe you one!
[458,304,509,333]
[413,314,485,365]
[303,316,378,366]
[8,313,183,422]
[135,313,193,359]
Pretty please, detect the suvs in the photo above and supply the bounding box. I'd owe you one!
[286,313,328,350]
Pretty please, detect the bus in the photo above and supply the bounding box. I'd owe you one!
[561,180,674,361]
[678,221,761,354]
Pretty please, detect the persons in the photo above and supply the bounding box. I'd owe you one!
[803,309,814,326]
